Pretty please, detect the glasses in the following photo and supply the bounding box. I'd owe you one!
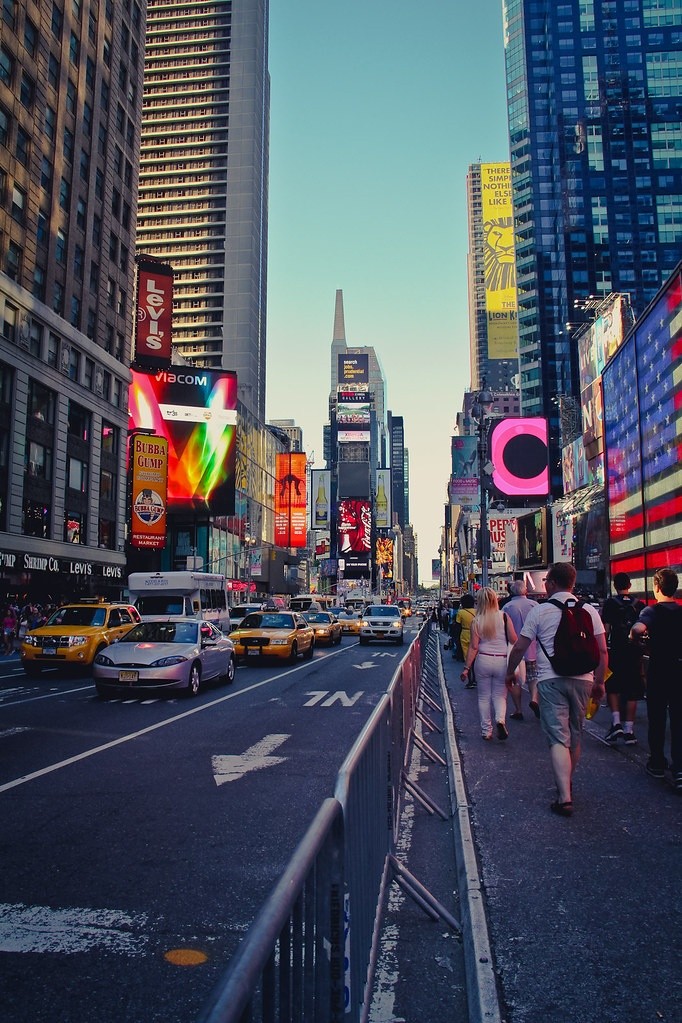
[655,568,662,580]
[542,578,557,583]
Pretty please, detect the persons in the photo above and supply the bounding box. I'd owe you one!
[499,580,540,720]
[627,568,682,797]
[505,562,607,815]
[602,572,646,745]
[454,550,462,588]
[0,599,70,656]
[436,594,478,689]
[460,587,518,741]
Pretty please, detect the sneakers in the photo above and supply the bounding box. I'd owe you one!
[605,721,624,742]
[672,770,682,791]
[623,732,638,744]
[645,761,665,778]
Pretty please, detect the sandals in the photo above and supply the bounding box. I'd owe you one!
[550,799,573,816]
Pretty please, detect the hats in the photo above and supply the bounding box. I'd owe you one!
[510,580,527,596]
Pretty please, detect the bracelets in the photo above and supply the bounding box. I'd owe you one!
[464,666,470,670]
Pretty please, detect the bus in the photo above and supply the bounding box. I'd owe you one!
[395,598,412,617]
[344,598,374,608]
[288,596,337,611]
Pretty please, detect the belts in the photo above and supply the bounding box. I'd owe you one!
[479,652,507,656]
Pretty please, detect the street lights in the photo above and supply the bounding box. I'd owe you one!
[471,376,495,587]
[244,531,257,603]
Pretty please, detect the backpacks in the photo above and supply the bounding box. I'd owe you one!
[608,595,640,648]
[536,598,600,677]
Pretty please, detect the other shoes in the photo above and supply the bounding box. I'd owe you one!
[465,682,477,689]
[529,701,540,719]
[496,722,508,740]
[482,735,492,741]
[510,713,524,721]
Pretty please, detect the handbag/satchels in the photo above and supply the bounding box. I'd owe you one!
[584,666,613,720]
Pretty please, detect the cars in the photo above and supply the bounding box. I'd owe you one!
[94,618,236,697]
[227,611,315,666]
[300,611,341,647]
[327,608,361,635]
[412,595,433,617]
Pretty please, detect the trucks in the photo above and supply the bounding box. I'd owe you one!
[128,571,230,636]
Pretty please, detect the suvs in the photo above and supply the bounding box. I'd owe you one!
[21,604,143,673]
[229,602,267,631]
[358,605,406,646]
